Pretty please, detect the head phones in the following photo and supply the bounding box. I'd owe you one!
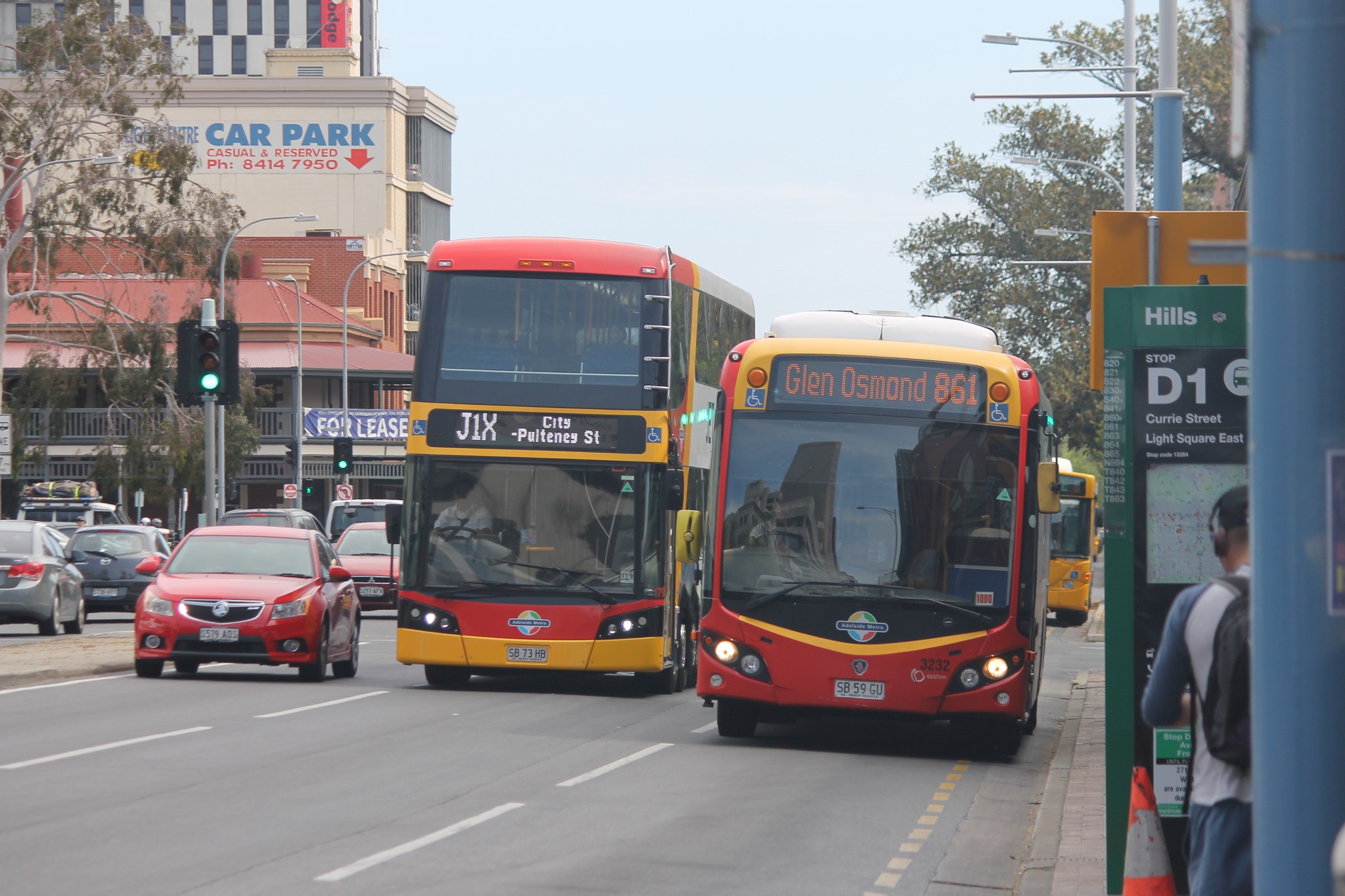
[1207,491,1229,556]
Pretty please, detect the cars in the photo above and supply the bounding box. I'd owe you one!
[335,522,404,611]
[135,525,361,681]
[0,519,174,636]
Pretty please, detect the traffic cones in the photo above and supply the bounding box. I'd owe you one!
[1122,767,1178,896]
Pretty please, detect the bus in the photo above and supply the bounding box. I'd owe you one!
[696,311,1099,739]
[396,235,756,692]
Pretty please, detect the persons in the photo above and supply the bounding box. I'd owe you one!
[1141,485,1256,896]
[94,512,114,525]
[432,479,493,538]
[73,516,87,535]
[151,518,162,528]
[607,322,627,346]
[141,517,151,526]
[749,488,799,548]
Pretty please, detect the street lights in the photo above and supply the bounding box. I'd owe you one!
[982,31,1139,213]
[342,250,429,485]
[1,155,123,217]
[283,273,304,510]
[218,214,320,521]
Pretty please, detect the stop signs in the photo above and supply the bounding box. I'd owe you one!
[285,485,297,498]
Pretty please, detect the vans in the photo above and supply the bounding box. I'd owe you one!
[217,508,343,544]
[325,499,419,551]
[16,498,136,527]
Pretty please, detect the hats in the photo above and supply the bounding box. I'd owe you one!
[1216,484,1249,529]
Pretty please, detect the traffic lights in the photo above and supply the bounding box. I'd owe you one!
[285,440,296,466]
[192,326,227,394]
[333,438,353,473]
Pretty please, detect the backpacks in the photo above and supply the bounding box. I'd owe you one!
[1186,576,1250,770]
